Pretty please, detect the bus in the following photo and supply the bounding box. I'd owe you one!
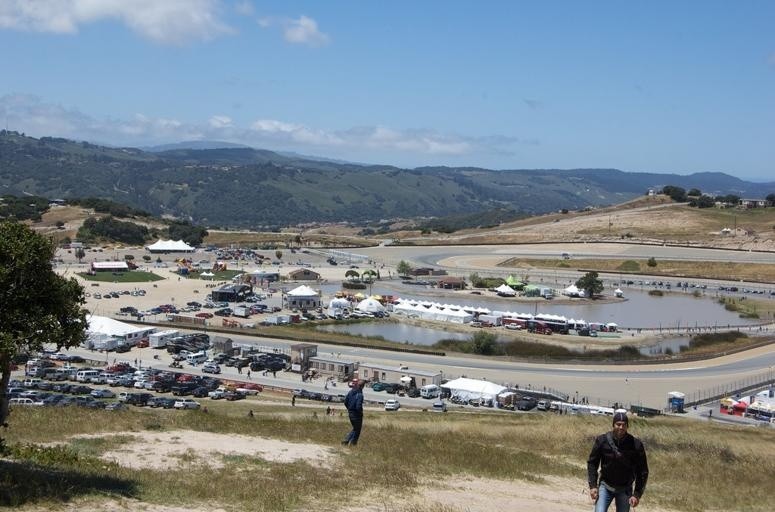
[718,399,774,422]
[85,287,148,300]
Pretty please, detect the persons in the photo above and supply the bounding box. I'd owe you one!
[247,369,250,379]
[341,379,366,448]
[248,410,253,417]
[238,365,241,374]
[326,406,331,415]
[313,411,317,419]
[134,358,138,364]
[292,395,296,406]
[587,412,649,512]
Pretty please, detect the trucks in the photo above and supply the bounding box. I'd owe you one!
[468,310,618,336]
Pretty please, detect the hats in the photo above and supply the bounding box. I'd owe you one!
[613,413,628,427]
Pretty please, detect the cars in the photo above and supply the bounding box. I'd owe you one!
[116,290,280,318]
[346,376,359,388]
[7,329,291,411]
[286,306,390,325]
[432,400,447,413]
[498,392,629,415]
[370,376,441,398]
[210,247,264,262]
[613,278,775,298]
[288,388,347,403]
[384,398,402,410]
